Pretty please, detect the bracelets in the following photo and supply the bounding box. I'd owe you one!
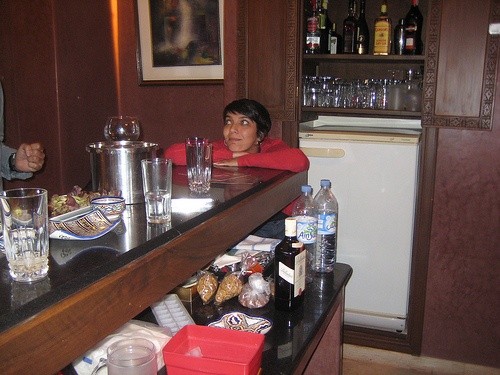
[10,153,17,170]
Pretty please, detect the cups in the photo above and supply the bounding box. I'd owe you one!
[302,69,422,112]
[106,337,158,375]
[187,137,208,143]
[0,187,50,284]
[140,158,173,224]
[103,116,140,147]
[185,143,212,194]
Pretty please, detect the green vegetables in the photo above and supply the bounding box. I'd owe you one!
[13,185,120,220]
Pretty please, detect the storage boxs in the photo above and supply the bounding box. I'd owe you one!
[162,325,264,375]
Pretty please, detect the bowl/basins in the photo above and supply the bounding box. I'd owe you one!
[90,196,126,221]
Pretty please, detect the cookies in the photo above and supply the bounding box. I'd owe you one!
[197,274,242,304]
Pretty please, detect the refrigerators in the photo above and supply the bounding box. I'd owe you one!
[297,117,422,330]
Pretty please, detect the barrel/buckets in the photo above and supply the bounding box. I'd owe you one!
[85,140,160,204]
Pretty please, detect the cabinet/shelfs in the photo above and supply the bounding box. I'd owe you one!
[301,0,428,117]
[0,168,353,375]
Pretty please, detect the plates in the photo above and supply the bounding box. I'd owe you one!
[50,206,95,222]
[205,311,274,335]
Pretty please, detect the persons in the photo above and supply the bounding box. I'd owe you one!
[0,83,46,197]
[160,98,313,219]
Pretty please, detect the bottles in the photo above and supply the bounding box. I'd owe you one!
[313,179,339,273]
[302,0,424,55]
[291,184,319,284]
[274,218,306,312]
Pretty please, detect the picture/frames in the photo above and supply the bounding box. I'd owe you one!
[134,0,224,86]
